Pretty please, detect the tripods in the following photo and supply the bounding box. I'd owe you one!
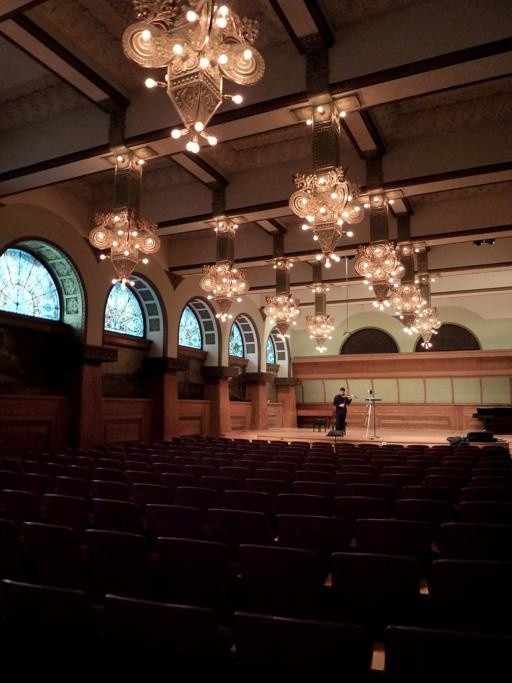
[362,402,379,439]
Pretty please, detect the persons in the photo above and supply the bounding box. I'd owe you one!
[332,386,354,431]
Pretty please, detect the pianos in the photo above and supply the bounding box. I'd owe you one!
[473,408,512,434]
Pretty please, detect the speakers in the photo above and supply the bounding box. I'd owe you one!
[467,431,494,442]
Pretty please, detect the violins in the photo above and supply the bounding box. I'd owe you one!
[342,395,358,399]
